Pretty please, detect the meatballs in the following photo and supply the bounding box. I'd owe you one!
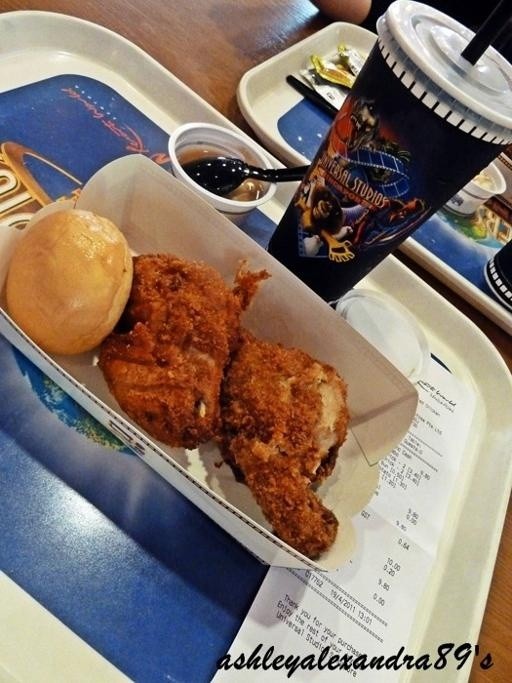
[8,208,134,357]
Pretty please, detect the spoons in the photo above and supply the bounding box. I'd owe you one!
[181,152,317,195]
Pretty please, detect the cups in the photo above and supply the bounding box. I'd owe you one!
[264,0,511,317]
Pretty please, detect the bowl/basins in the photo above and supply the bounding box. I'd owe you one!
[448,163,508,214]
[165,122,278,223]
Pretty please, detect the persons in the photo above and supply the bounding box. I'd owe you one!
[310,0,512,64]
